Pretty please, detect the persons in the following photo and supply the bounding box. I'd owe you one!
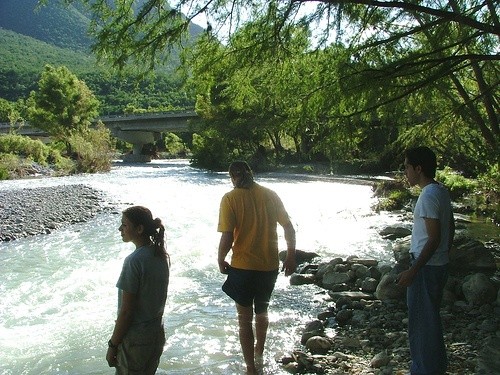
[103,206,169,375]
[216,160,297,375]
[394,147,455,374]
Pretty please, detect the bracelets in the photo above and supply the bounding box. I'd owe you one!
[108,340,118,348]
